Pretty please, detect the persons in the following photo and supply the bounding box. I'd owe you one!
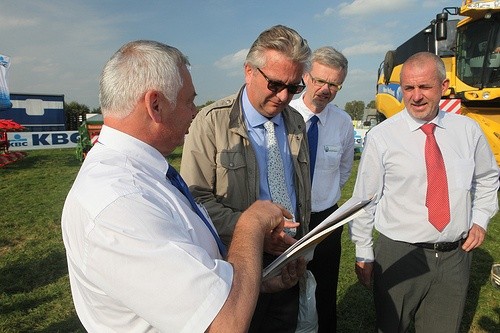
[62,40,295,333]
[178,24,311,333]
[351,51,500,333]
[286,46,354,333]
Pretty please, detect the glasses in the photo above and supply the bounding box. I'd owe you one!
[307,71,342,91]
[256,66,306,96]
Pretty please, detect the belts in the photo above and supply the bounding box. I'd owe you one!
[310,204,338,225]
[396,237,468,253]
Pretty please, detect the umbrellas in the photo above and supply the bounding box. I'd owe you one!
[0,118,29,153]
[0,53,13,109]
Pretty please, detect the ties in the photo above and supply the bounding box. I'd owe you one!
[306,115,318,186]
[420,122,451,233]
[167,163,227,262]
[264,120,297,238]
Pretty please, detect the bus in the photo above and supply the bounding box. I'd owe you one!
[363,0,500,170]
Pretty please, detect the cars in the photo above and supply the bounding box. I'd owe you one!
[352,119,373,153]
